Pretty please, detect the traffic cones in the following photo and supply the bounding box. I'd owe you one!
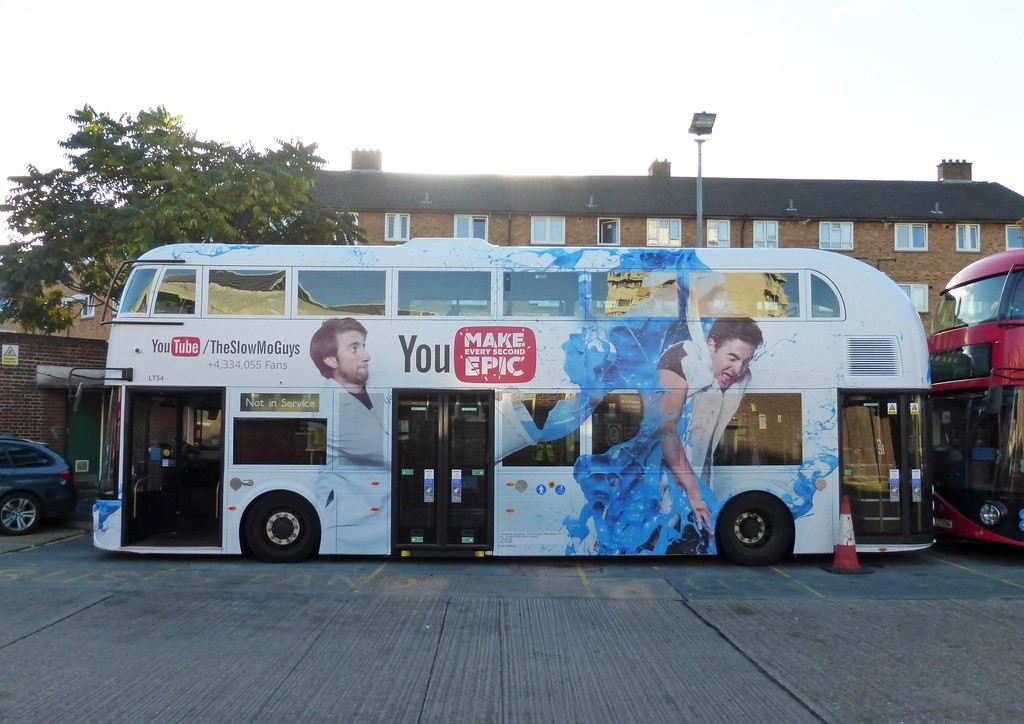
[820,493,875,575]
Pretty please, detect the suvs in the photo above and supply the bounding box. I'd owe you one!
[0,434,79,538]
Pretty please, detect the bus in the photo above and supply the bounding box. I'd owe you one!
[924,245,1024,560]
[67,234,930,566]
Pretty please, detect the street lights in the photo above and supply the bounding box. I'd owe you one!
[687,111,717,249]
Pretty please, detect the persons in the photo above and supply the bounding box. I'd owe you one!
[310,316,385,467]
[656,316,763,533]
